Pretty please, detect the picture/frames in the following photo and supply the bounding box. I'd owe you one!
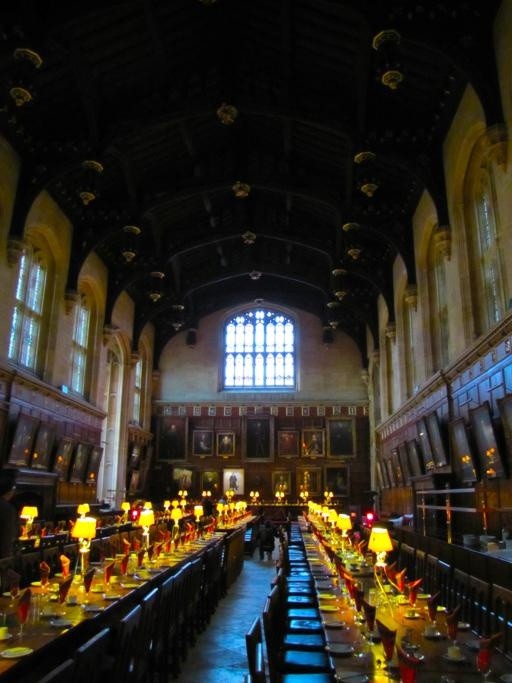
[126,413,358,498]
[375,390,509,489]
[9,411,104,486]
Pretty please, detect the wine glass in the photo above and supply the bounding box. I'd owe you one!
[335,552,495,682]
[0,536,172,655]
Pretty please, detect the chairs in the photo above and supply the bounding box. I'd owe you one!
[2,493,264,683]
[241,495,509,683]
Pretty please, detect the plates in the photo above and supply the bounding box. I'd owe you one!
[1,648,33,659]
